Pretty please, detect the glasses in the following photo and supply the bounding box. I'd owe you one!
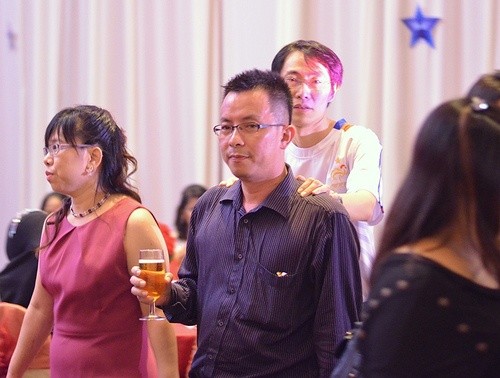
[42,143,104,158]
[213,121,288,136]
[471,98,491,113]
[284,74,336,90]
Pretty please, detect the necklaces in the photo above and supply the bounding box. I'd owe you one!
[69,189,110,219]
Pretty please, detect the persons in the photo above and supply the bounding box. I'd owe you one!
[130,67,364,378]
[6,104,180,378]
[270,40,384,300]
[328,98,500,377]
[168,184,208,266]
[0,191,71,309]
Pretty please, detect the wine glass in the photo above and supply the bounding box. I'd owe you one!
[139,249,166,321]
